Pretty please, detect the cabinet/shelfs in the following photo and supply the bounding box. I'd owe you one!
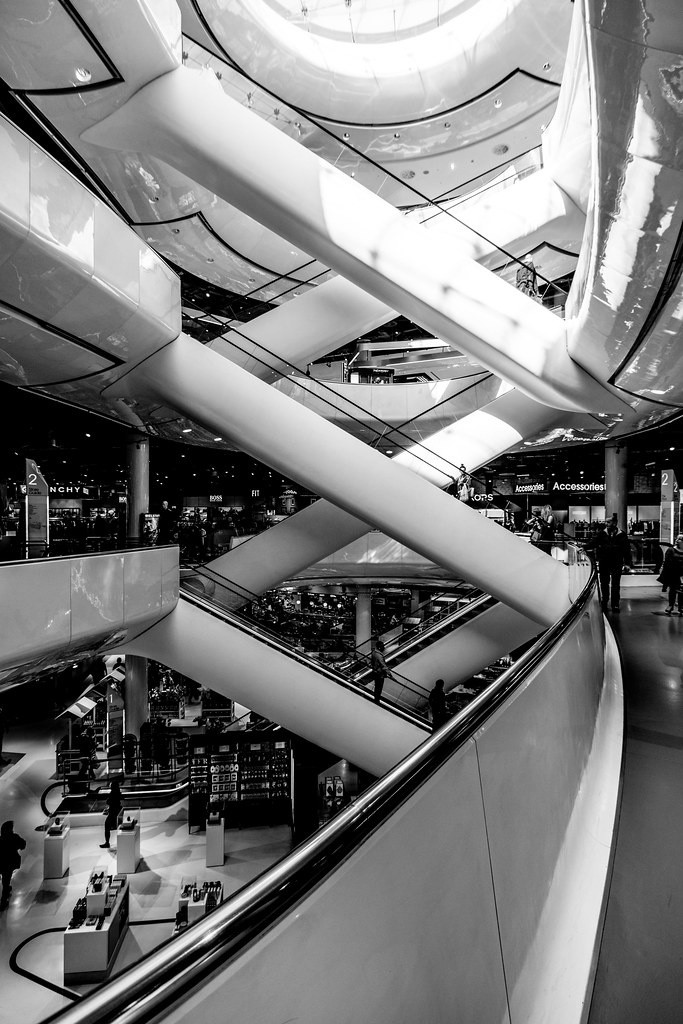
[187,732,297,835]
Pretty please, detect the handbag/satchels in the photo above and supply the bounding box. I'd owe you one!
[530,531,541,542]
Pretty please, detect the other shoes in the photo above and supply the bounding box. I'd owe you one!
[100,843,110,848]
[2,886,12,895]
[613,606,619,613]
[678,608,683,612]
[665,605,674,612]
[602,598,609,605]
[0,901,9,912]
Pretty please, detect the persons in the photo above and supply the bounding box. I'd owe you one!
[578,517,631,614]
[100,780,125,848]
[371,640,393,704]
[156,500,175,546]
[570,519,588,524]
[0,821,26,911]
[429,679,446,733]
[112,658,126,670]
[657,534,683,614]
[525,505,555,556]
[148,661,177,685]
[78,727,101,778]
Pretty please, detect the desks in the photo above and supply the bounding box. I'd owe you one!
[171,881,224,936]
[63,879,132,987]
